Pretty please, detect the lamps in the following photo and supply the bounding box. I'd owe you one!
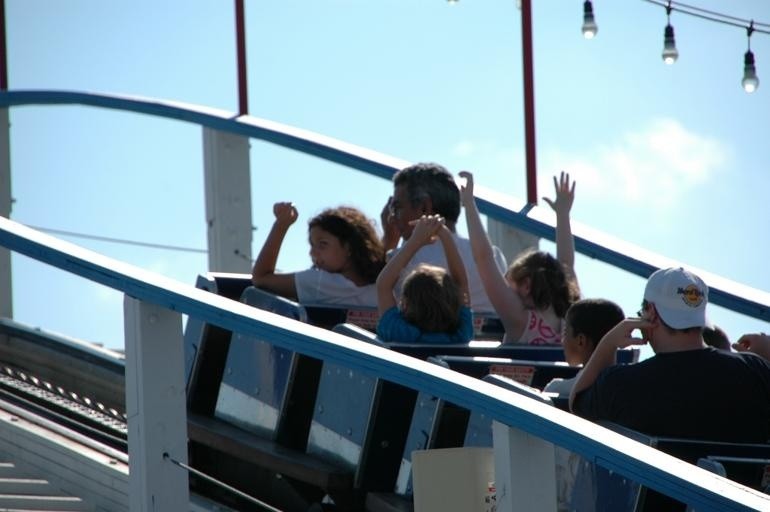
[580,0,598,38]
[662,0,679,65]
[741,19,759,94]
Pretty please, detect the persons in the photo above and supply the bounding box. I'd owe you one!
[702,325,731,352]
[568,267,770,443]
[380,163,510,312]
[376,214,473,347]
[252,202,386,309]
[544,298,626,393]
[459,172,578,346]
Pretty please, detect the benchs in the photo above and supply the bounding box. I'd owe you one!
[184,272,770,511]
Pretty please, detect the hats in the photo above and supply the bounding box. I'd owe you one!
[644,267,708,330]
